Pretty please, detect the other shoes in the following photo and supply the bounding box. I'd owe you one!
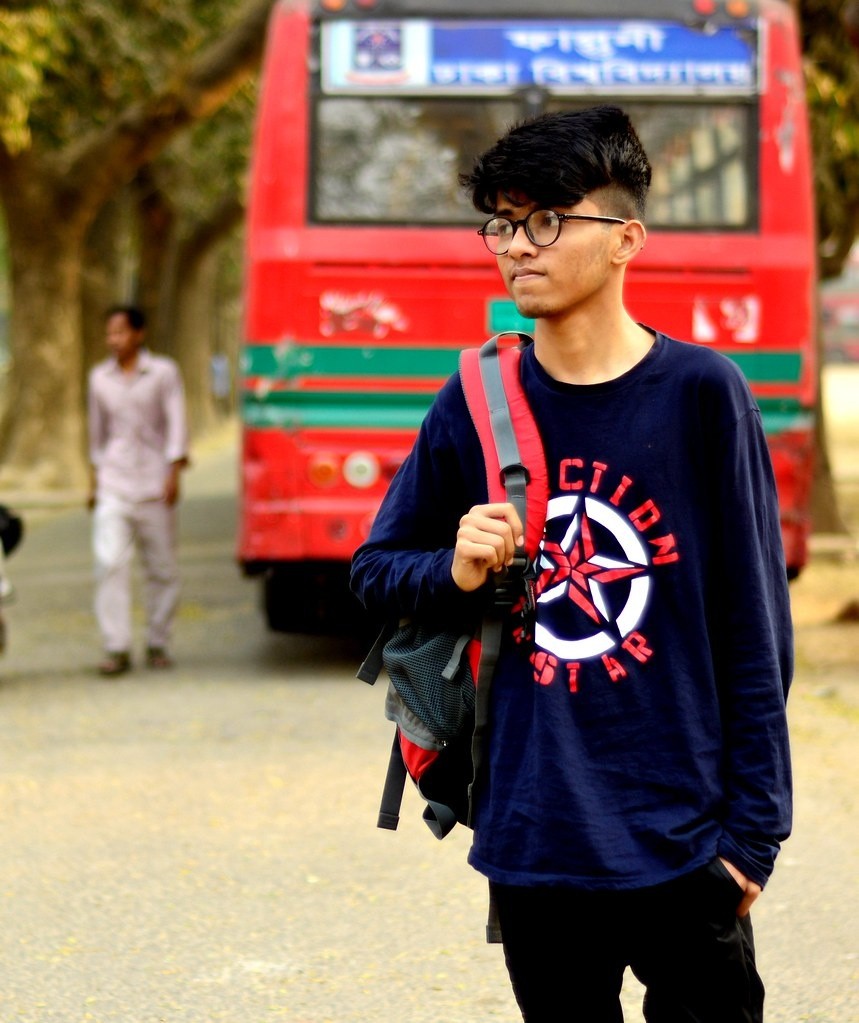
[101,653,127,674]
[148,649,170,668]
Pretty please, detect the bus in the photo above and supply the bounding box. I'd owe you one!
[235,1,817,634]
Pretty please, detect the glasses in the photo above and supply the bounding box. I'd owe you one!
[477,207,643,255]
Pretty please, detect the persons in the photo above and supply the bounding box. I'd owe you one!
[83,304,191,675]
[349,105,795,1023]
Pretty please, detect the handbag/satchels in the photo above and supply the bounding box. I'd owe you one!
[354,332,549,841]
